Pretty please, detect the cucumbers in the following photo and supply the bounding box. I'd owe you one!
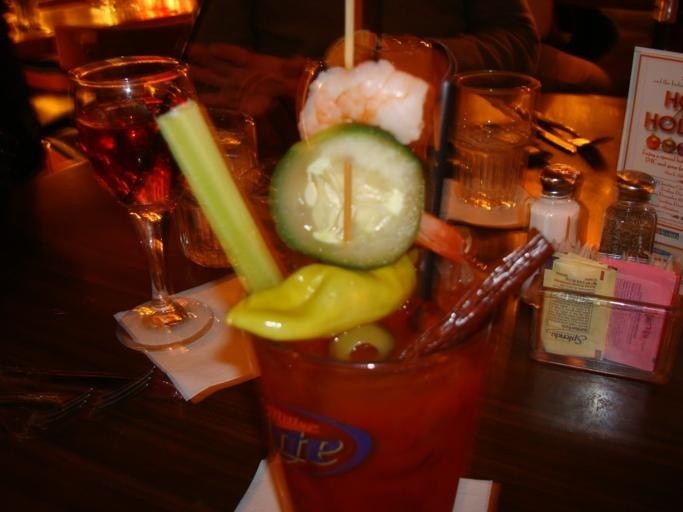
[266,122,425,270]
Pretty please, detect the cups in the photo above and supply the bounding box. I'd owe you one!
[239,295,504,512]
[175,104,259,270]
[449,68,543,215]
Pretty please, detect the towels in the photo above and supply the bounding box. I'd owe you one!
[114,273,259,404]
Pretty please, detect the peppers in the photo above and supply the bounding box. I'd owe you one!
[225,252,417,341]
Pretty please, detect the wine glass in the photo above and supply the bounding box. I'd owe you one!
[64,54,214,353]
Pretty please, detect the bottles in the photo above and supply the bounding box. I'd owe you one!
[517,163,583,311]
[597,168,658,261]
